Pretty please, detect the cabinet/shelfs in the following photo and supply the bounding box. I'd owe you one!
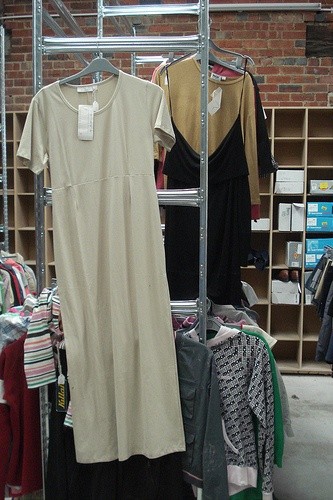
[1,111,55,288]
[240,106,333,375]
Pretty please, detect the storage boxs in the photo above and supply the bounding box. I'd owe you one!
[285,241,303,268]
[278,203,290,232]
[307,201,333,231]
[308,180,332,194]
[273,170,304,193]
[305,239,332,267]
[272,280,300,303]
[291,203,304,231]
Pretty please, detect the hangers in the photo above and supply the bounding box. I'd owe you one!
[59,38,118,85]
[159,18,255,76]
[195,298,223,334]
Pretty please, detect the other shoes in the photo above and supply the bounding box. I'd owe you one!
[277,269,289,281]
[291,270,299,282]
